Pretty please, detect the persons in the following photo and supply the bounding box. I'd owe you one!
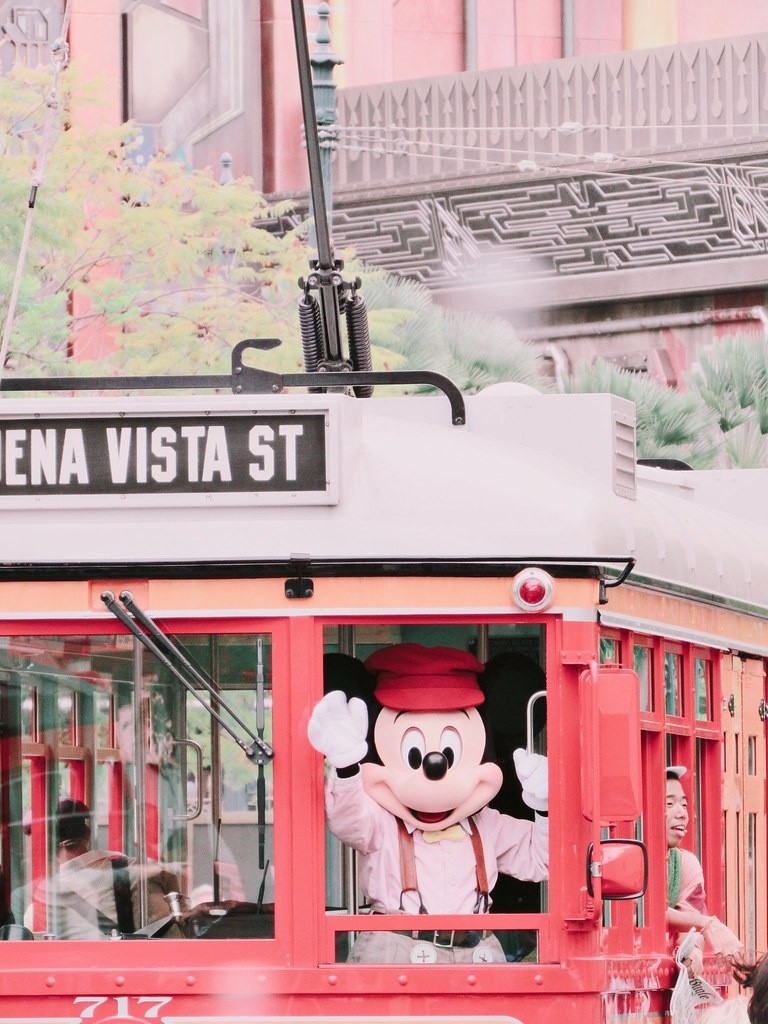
[34,701,242,940]
[666,766,748,971]
[10,797,183,940]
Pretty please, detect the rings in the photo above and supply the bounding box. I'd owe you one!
[714,951,722,956]
[727,953,733,957]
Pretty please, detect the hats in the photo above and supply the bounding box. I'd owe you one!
[666,767,687,778]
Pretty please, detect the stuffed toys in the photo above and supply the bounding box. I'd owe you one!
[308,643,549,964]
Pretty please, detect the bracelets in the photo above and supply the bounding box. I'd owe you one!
[700,915,716,934]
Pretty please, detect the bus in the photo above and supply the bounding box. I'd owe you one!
[0,0,766,1021]
